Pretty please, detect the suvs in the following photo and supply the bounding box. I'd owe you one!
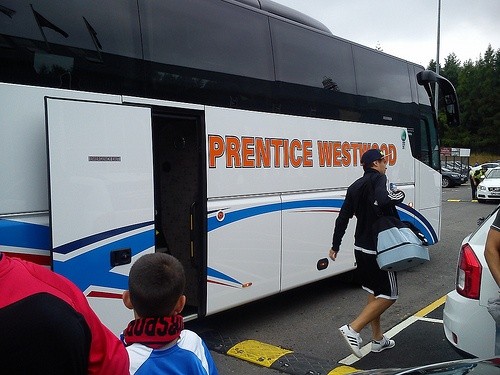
[443,205,500,366]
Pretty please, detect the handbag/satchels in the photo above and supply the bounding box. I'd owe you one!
[373,215,430,272]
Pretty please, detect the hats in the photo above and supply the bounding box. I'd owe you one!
[360,148,392,166]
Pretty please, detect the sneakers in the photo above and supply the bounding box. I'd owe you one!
[370,335,396,353]
[339,323,362,358]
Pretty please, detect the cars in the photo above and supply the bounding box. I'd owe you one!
[441,168,463,188]
[441,161,469,184]
[477,168,500,199]
[469,163,500,179]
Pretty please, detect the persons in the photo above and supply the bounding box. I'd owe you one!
[119,252,220,375]
[0,250,131,375]
[328,148,406,359]
[470,165,488,200]
[483,209,500,289]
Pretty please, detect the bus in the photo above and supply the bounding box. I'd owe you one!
[0,0,461,340]
